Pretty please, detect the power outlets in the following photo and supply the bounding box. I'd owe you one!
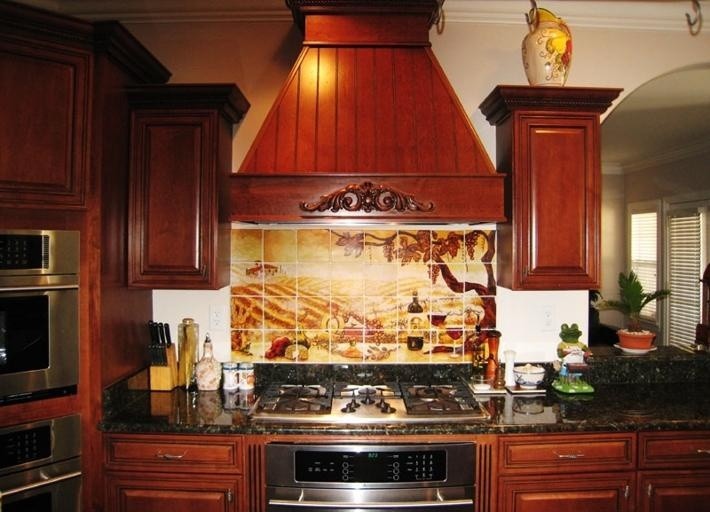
[209,304,227,332]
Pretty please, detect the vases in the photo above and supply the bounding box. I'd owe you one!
[523,0,572,88]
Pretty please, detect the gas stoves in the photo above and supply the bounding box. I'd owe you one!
[247,383,493,426]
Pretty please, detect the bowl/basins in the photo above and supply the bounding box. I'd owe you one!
[512,399,545,416]
[470,374,494,391]
[513,366,547,390]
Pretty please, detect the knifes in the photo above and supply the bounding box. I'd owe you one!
[148,322,172,366]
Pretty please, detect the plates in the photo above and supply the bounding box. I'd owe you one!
[613,343,658,356]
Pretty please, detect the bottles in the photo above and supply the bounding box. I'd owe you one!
[177,318,199,390]
[221,362,238,391]
[495,361,505,390]
[407,291,423,351]
[234,363,255,389]
[196,389,223,427]
[233,389,255,409]
[194,335,221,391]
[223,390,237,411]
[504,350,516,386]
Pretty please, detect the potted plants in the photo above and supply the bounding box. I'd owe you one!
[589,271,669,356]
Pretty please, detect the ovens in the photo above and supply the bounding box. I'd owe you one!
[0,274,81,407]
[264,485,477,512]
[0,455,83,512]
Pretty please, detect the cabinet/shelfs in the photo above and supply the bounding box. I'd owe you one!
[478,86,624,291]
[125,82,251,290]
[0,0,91,229]
[102,434,252,512]
[492,431,710,511]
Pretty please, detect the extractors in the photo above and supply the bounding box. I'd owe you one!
[220,0,507,226]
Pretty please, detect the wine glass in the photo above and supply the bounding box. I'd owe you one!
[445,316,461,358]
[425,300,447,353]
